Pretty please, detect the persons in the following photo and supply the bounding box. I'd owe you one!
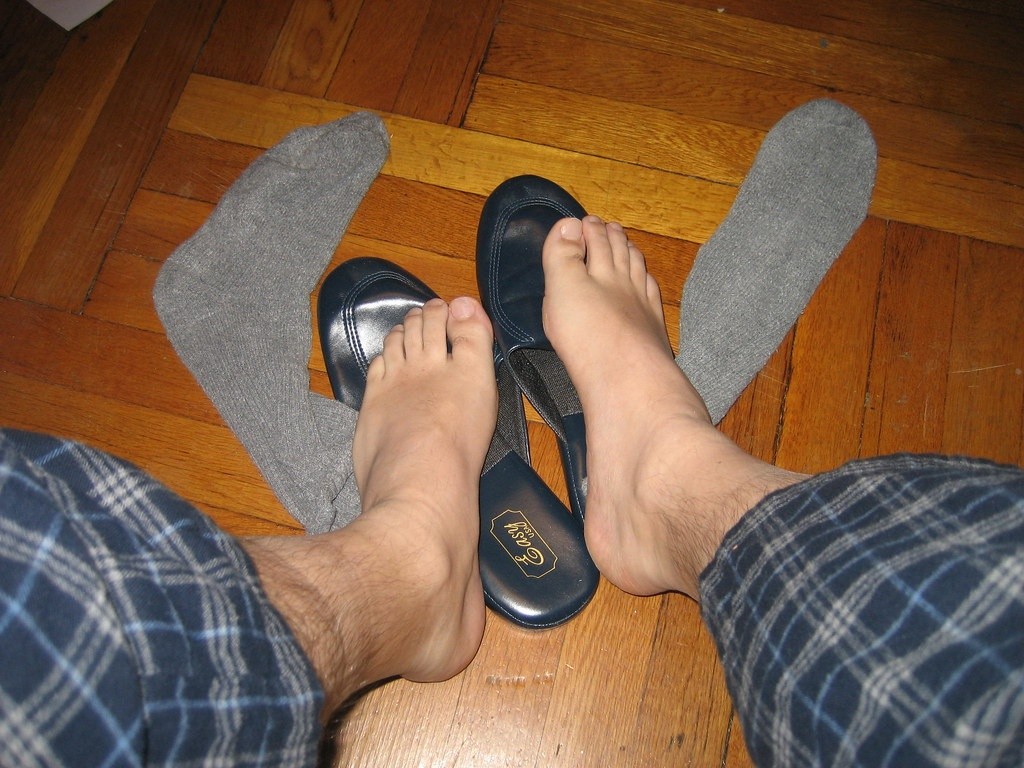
[0,212,1024,768]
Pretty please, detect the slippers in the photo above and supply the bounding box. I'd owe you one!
[317,256,600,629]
[475,176,590,530]
